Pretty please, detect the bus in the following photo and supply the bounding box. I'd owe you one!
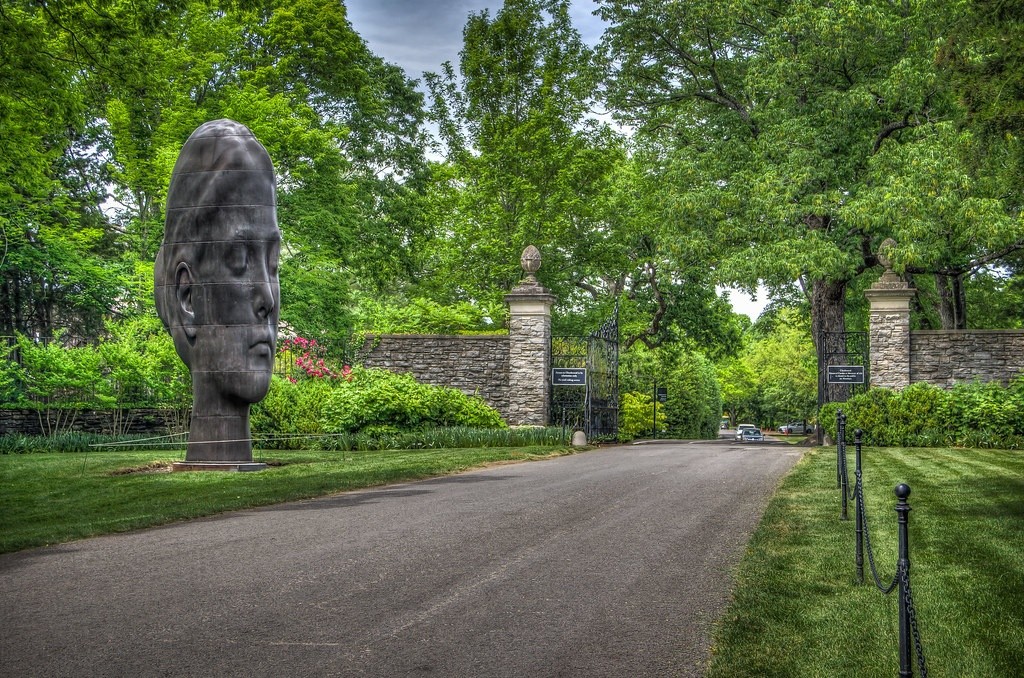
[721,415,730,425]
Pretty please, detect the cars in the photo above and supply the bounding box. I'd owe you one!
[720,421,730,429]
[779,421,814,435]
[734,424,766,444]
